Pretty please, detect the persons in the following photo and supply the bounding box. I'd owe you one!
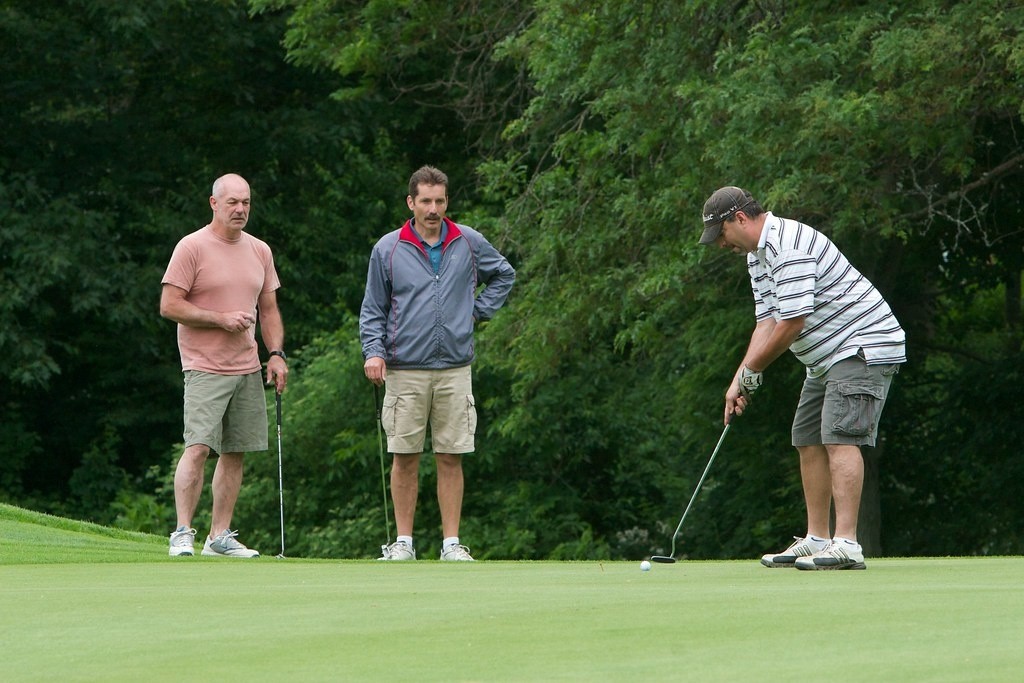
[359,165,516,560]
[698,186,907,571]
[159,174,289,559]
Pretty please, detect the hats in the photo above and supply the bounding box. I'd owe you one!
[697,186,754,244]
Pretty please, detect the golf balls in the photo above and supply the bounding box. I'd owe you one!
[641,560,651,571]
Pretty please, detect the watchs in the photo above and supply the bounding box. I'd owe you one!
[269,351,286,361]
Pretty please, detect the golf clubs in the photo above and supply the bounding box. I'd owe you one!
[650,391,743,563]
[273,372,286,560]
[373,381,393,559]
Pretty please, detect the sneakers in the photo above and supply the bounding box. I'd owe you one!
[440,543,473,561]
[200,529,260,559]
[169,526,194,556]
[761,535,867,571]
[377,541,417,561]
